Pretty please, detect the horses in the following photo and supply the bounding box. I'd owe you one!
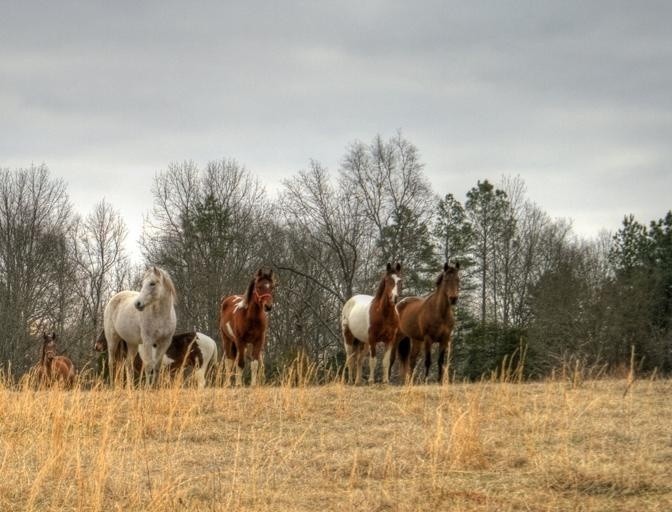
[95,330,218,392]
[395,260,461,385]
[341,262,403,387]
[103,261,179,390]
[38,330,75,394]
[219,268,275,387]
